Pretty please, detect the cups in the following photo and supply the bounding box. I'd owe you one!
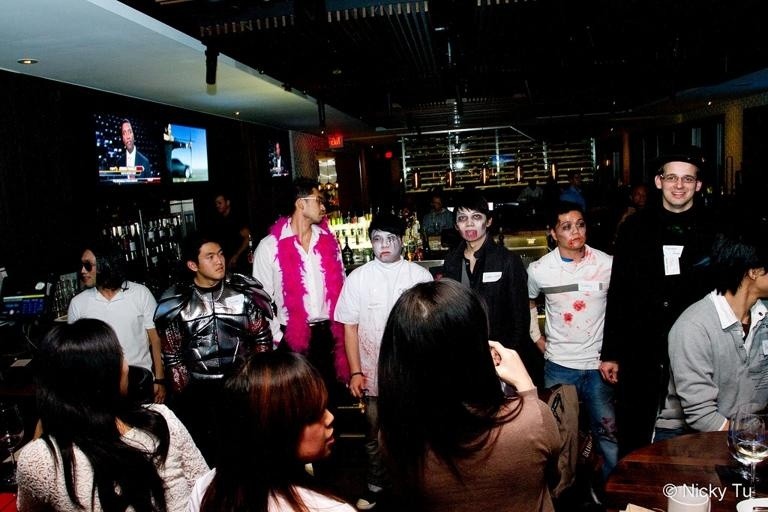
[668,485,712,511]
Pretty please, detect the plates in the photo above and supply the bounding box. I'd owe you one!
[734,497,768,512]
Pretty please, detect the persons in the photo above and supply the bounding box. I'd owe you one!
[114,118,151,179]
[162,122,193,183]
[14,145,768,512]
[273,142,289,177]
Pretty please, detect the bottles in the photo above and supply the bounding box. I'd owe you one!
[319,204,425,266]
[497,227,504,246]
[549,162,559,181]
[100,214,186,267]
[52,274,79,311]
[412,171,421,190]
[480,166,491,185]
[445,168,455,187]
[514,164,524,183]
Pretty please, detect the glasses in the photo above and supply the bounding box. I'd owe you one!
[79,263,97,273]
[660,174,697,183]
[301,197,324,204]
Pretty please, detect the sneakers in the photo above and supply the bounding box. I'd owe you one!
[356,485,381,509]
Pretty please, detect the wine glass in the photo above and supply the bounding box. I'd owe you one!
[724,403,768,503]
[0,400,25,486]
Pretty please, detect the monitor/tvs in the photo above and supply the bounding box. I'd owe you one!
[92,108,159,187]
[266,135,292,179]
[159,119,212,186]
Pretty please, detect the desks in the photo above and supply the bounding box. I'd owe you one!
[605,430,768,512]
